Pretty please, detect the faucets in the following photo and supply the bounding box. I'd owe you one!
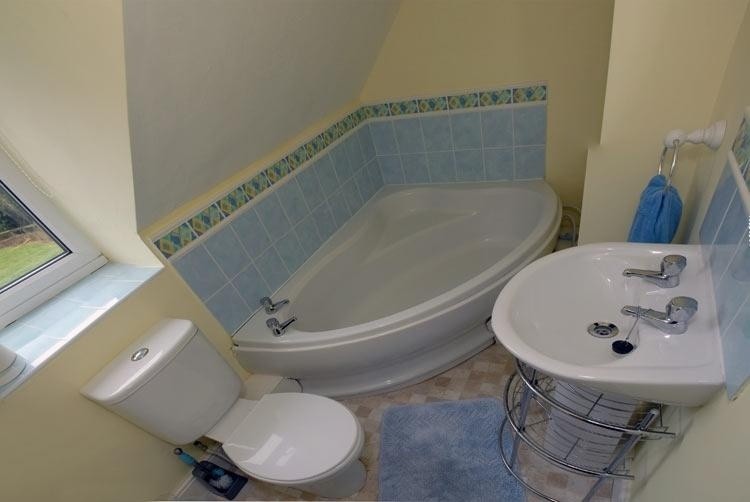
[621,295,698,335]
[265,312,297,335]
[262,296,290,313]
[621,255,688,289]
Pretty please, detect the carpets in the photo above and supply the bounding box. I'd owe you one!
[378,398,527,502]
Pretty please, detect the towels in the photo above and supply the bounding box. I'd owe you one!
[628,175,682,244]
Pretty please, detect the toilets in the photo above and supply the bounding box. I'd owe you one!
[79,319,365,499]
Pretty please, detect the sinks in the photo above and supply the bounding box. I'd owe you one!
[491,241,726,408]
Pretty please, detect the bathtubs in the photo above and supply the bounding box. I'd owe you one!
[229,180,562,400]
